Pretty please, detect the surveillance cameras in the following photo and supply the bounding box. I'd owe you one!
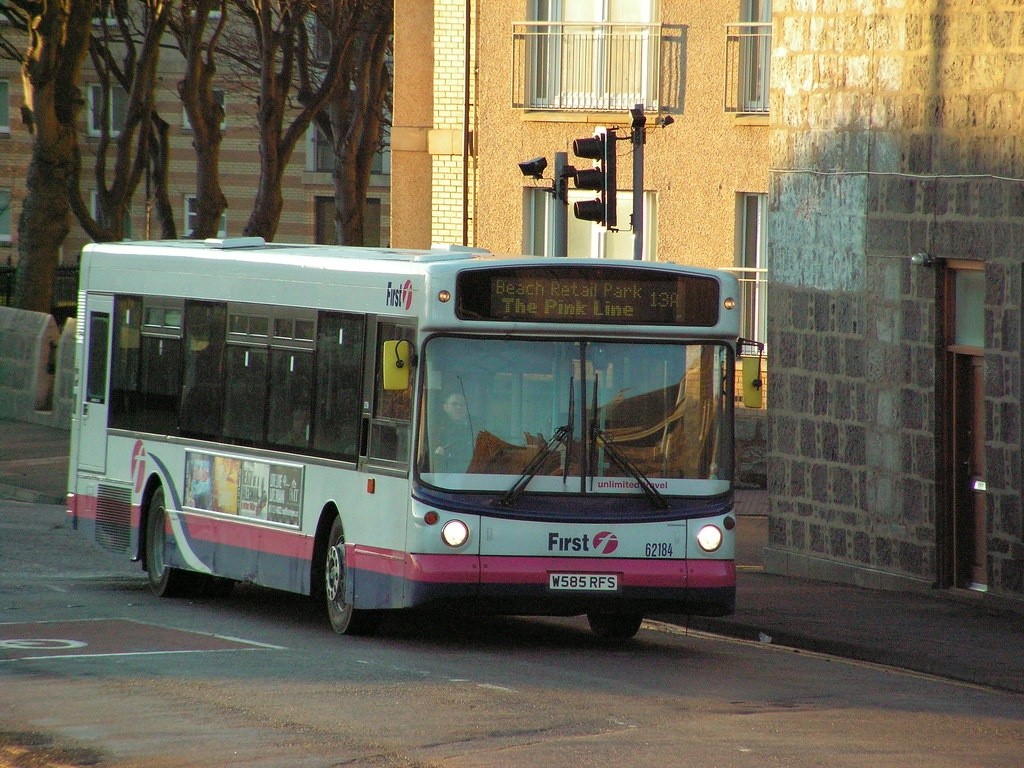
[519,157,547,176]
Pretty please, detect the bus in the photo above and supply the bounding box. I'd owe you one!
[64,234,765,648]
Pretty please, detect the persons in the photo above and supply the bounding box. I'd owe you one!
[431,393,488,471]
[270,377,311,445]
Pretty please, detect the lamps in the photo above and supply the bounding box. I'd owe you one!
[910,252,930,266]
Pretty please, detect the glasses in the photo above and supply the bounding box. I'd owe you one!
[446,401,467,406]
[293,415,310,421]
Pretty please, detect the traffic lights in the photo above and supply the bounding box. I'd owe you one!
[573,126,618,229]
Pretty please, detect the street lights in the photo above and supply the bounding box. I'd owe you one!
[518,154,567,257]
